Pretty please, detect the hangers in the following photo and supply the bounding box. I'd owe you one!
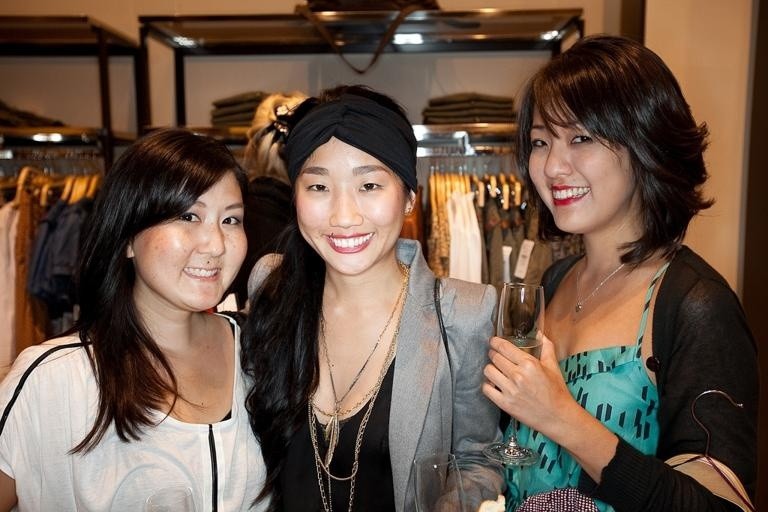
[429,145,525,222]
[673,388,756,512]
[10,146,103,210]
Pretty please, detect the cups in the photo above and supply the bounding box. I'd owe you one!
[146,486,197,512]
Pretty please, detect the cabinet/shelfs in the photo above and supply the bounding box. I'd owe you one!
[0,16,140,176]
[136,8,587,147]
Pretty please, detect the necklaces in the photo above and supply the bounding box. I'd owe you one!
[306,261,410,511]
[574,263,625,312]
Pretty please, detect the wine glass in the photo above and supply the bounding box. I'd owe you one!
[481,282,547,467]
[411,452,468,512]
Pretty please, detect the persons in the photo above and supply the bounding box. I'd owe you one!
[240,83,505,512]
[481,33,759,512]
[0,131,272,512]
